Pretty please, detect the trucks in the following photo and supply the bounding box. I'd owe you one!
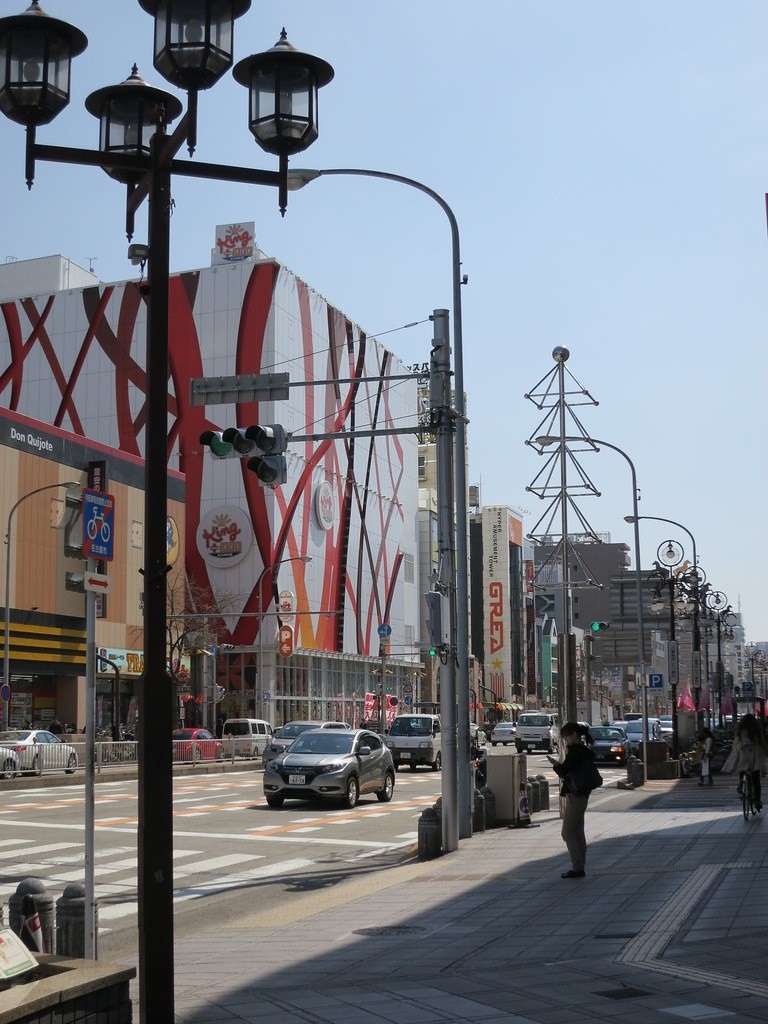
[386,713,476,771]
[515,712,559,755]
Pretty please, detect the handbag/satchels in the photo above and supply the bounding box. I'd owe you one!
[588,767,603,789]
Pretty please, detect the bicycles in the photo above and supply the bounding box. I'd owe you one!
[0,721,75,735]
[736,769,767,820]
[96,722,135,742]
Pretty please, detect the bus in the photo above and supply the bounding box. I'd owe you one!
[624,712,642,722]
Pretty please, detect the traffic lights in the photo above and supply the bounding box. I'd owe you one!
[591,622,609,632]
[199,424,288,460]
[429,648,437,656]
[247,456,287,487]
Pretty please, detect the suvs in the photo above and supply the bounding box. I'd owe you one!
[261,720,352,771]
[263,730,396,809]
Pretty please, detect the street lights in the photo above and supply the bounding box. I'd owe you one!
[536,434,650,786]
[623,514,768,759]
[1,1,335,1024]
[257,554,311,718]
[0,480,82,730]
[284,166,479,841]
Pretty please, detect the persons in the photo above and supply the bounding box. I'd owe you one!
[48,716,63,734]
[548,721,593,878]
[721,713,767,812]
[21,720,33,730]
[698,727,714,786]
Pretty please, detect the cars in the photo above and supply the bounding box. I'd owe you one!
[577,715,674,768]
[455,723,488,746]
[490,722,519,746]
[172,728,225,764]
[0,746,20,779]
[0,730,79,777]
[274,726,298,734]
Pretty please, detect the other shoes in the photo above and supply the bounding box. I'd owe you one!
[736,784,743,793]
[561,870,586,877]
[754,802,762,808]
[698,781,704,785]
[709,781,713,786]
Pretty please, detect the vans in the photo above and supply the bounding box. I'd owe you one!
[223,718,276,761]
